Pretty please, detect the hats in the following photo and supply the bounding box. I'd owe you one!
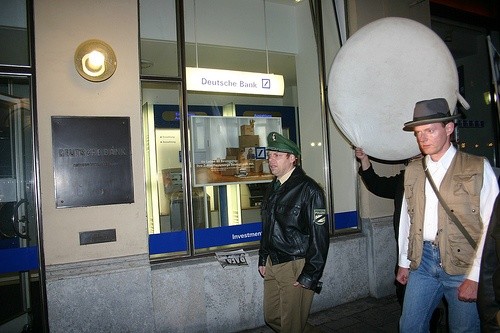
[404,97,461,128]
[265,131,302,158]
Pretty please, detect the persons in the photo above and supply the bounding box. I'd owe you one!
[396,98,499,333]
[257,132,331,333]
[354,146,407,304]
